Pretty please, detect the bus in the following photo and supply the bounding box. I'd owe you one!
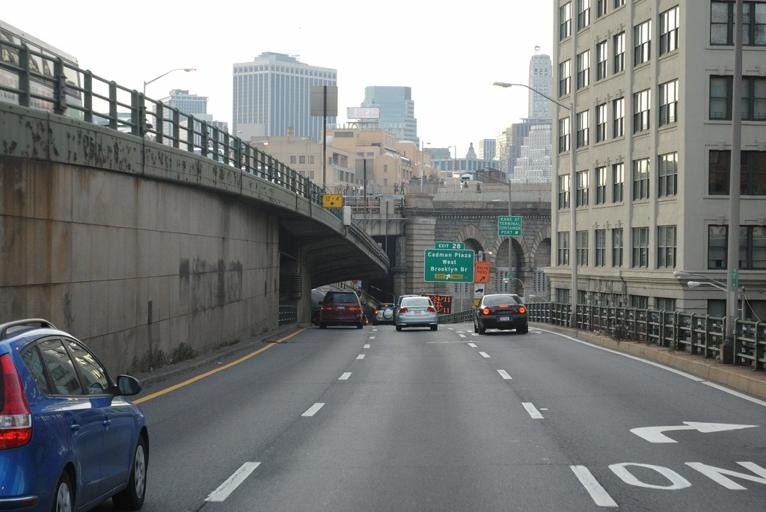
[0,19,82,121]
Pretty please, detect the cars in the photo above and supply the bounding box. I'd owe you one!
[472,294,528,335]
[319,289,437,331]
[0,317,149,512]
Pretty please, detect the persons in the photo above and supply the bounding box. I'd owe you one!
[392,182,399,195]
[459,180,464,190]
[344,183,349,195]
[400,181,405,195]
[352,184,357,195]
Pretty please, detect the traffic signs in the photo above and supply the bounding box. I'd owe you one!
[424,240,474,284]
[498,215,523,236]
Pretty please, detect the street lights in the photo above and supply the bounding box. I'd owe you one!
[504,277,526,296]
[144,69,196,96]
[422,142,431,168]
[529,295,549,302]
[449,146,456,171]
[453,171,512,293]
[493,82,578,327]
[687,281,747,321]
